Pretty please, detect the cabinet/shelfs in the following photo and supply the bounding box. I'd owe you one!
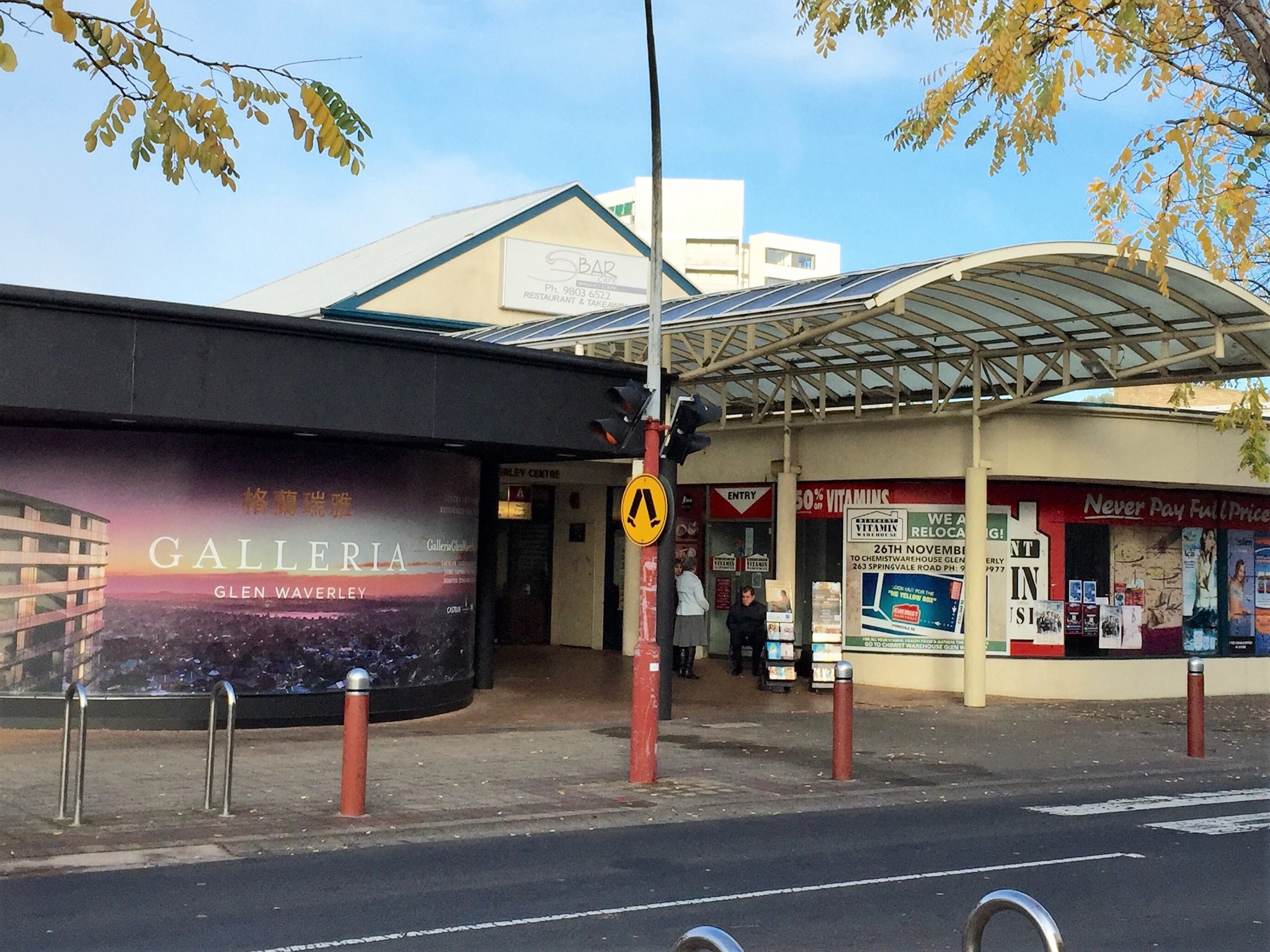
[756,617,799,694]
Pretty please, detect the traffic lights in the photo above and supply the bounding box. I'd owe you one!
[658,397,722,468]
[659,367,681,398]
[596,385,658,448]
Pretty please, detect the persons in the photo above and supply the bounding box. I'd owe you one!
[672,557,710,680]
[1036,527,1250,636]
[726,586,768,677]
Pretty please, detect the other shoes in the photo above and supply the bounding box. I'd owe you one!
[751,668,761,676]
[731,666,743,676]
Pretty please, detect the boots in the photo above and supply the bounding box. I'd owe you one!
[678,648,687,677]
[685,647,699,679]
